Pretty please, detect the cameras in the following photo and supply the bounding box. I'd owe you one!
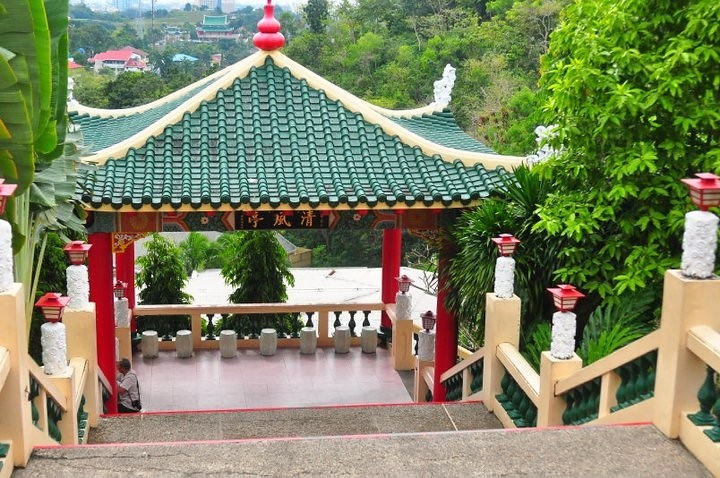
[132,400,142,410]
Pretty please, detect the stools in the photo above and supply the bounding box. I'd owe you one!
[360,326,378,353]
[142,330,158,357]
[175,330,194,358]
[220,330,237,357]
[333,326,351,354]
[258,328,277,355]
[300,327,317,354]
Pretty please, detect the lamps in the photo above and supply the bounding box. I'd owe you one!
[547,283,585,313]
[394,274,415,293]
[0,178,18,216]
[34,292,71,323]
[63,241,94,266]
[491,233,521,259]
[680,171,720,212]
[419,311,436,332]
[113,280,128,300]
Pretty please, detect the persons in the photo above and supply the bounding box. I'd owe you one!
[117,358,140,413]
